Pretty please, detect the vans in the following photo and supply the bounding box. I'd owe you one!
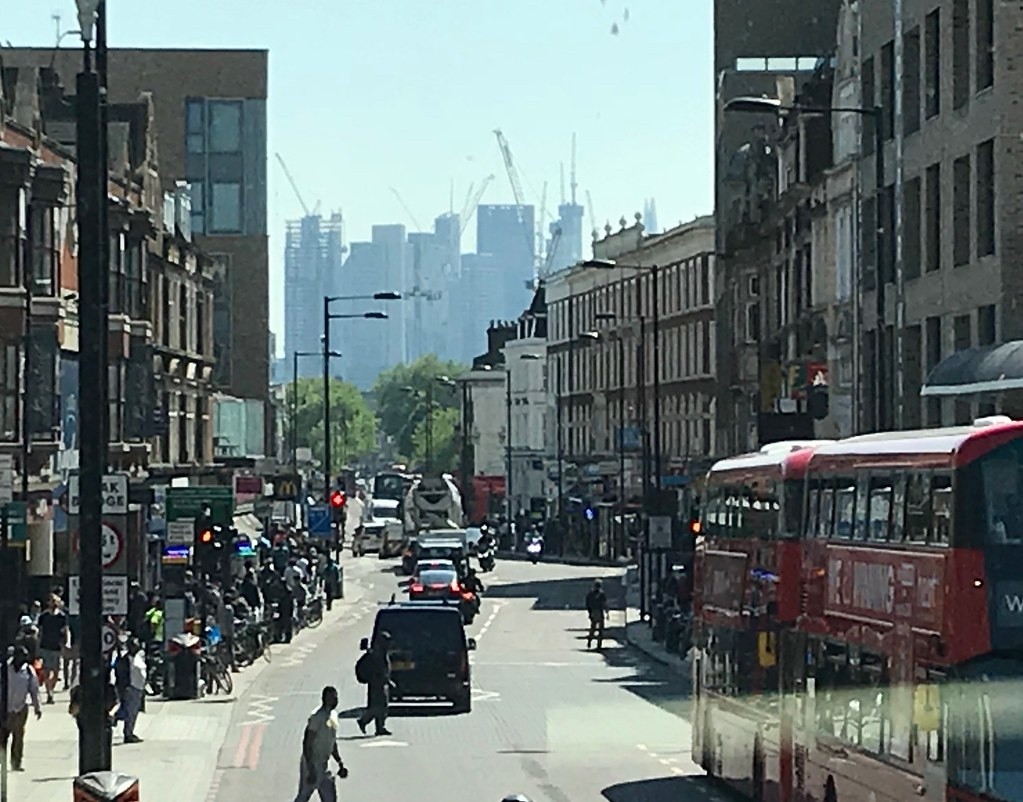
[419,530,467,558]
[360,497,402,526]
[360,602,476,712]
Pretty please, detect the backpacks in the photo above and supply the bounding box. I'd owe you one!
[355,653,374,684]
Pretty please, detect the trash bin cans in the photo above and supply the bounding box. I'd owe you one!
[166,633,201,698]
[72,771,140,802]
[334,565,343,599]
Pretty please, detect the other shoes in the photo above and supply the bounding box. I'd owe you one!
[357,720,366,734]
[375,728,391,736]
[124,735,144,743]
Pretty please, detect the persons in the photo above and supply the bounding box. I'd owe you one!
[524,523,544,551]
[584,578,611,652]
[293,686,349,802]
[358,632,400,737]
[6,645,43,772]
[476,531,495,567]
[112,636,148,744]
[7,529,343,705]
[458,568,484,614]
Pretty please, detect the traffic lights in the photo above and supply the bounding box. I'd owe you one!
[332,493,345,512]
[198,518,215,552]
[807,362,831,420]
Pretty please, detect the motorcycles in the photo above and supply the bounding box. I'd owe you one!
[523,538,542,564]
[478,538,497,570]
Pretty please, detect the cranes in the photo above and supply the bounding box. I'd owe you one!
[268,128,580,311]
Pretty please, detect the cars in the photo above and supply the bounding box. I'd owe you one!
[350,523,384,558]
[411,559,459,584]
[379,522,403,560]
[408,570,462,606]
[418,539,469,580]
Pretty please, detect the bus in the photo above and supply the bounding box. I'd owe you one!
[677,415,1023,802]
[374,471,410,501]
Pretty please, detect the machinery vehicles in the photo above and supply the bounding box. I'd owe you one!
[396,472,466,575]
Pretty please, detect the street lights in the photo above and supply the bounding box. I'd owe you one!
[437,376,467,474]
[580,334,627,556]
[580,261,662,608]
[293,346,342,475]
[401,387,432,471]
[322,290,403,504]
[477,365,512,551]
[597,311,654,619]
[725,95,887,433]
[522,352,565,553]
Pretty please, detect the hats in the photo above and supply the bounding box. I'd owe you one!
[382,631,392,640]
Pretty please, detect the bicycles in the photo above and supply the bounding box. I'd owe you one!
[144,583,324,694]
